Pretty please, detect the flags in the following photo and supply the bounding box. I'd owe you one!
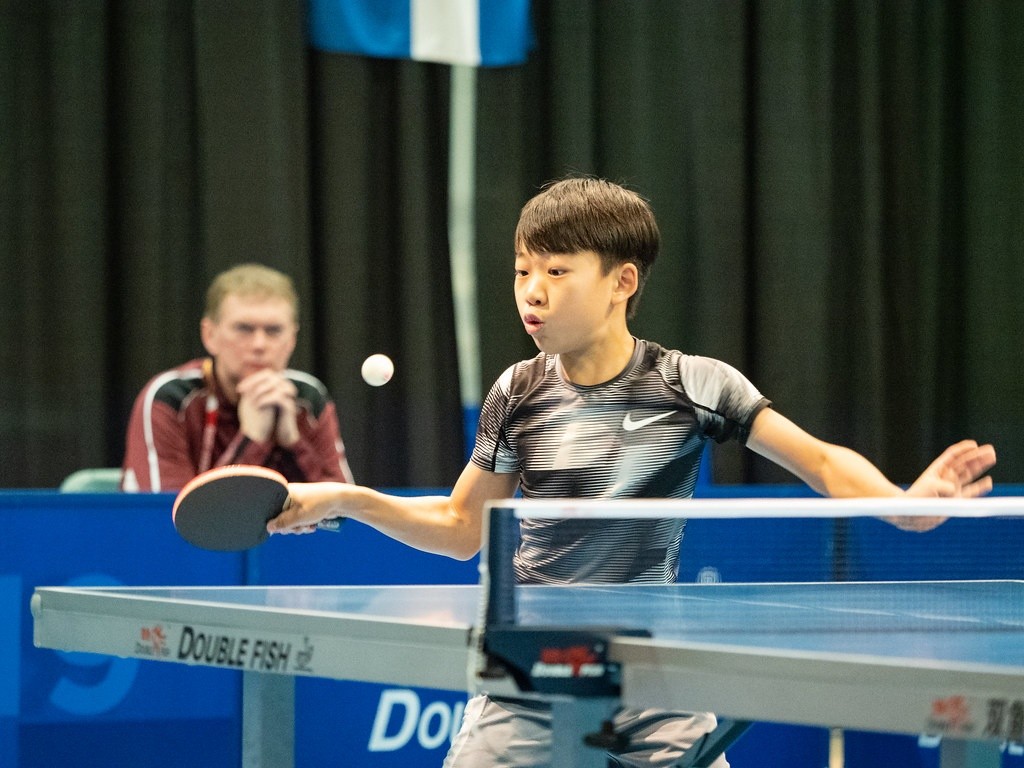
[309,0,534,68]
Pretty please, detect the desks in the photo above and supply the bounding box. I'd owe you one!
[27,495,1024,768]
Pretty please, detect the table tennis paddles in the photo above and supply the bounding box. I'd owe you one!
[171,463,345,554]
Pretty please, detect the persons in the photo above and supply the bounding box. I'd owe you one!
[267,180,996,768]
[118,265,356,495]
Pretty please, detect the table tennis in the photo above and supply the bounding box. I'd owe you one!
[361,353,395,387]
